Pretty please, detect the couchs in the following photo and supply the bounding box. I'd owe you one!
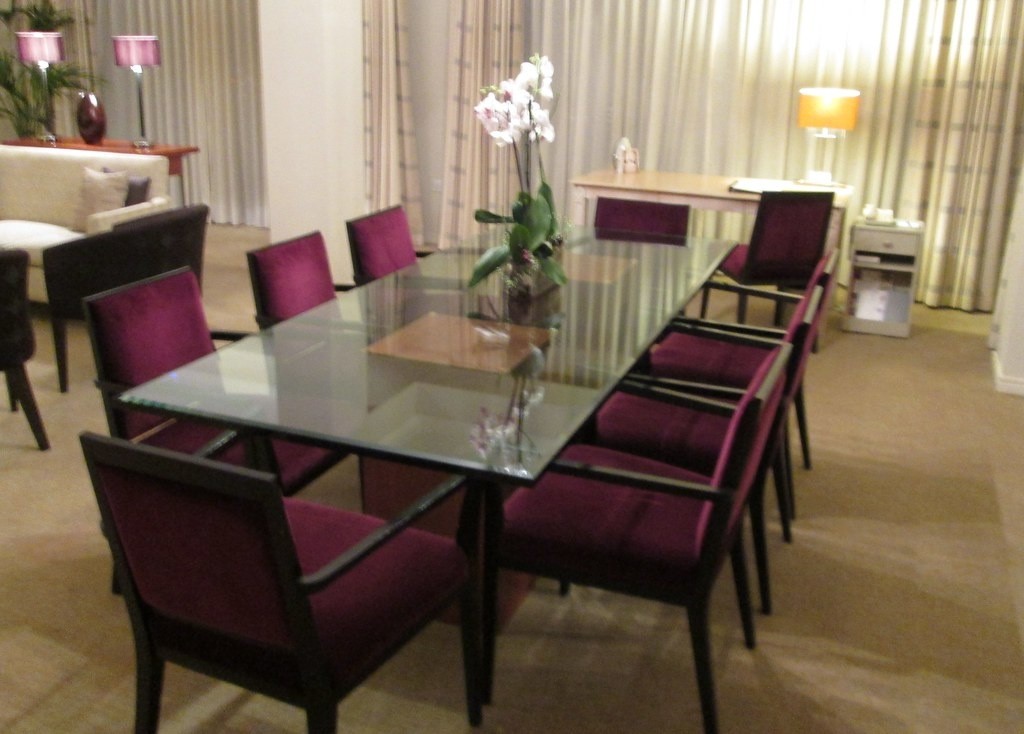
[1,145,179,305]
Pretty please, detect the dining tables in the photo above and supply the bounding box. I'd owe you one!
[115,240,742,703]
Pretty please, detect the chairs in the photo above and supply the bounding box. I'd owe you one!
[456,341,797,734]
[75,426,466,734]
[643,248,841,506]
[698,190,835,326]
[1,248,52,452]
[44,203,209,394]
[246,230,337,330]
[594,195,691,249]
[577,276,824,614]
[81,263,351,597]
[343,205,420,286]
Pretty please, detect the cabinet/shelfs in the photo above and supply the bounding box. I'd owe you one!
[840,220,924,339]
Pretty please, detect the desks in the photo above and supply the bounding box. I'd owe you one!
[1,135,200,207]
[568,167,859,310]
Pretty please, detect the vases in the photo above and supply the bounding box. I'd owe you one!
[505,234,566,301]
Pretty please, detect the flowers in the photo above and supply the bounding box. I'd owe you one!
[474,52,556,194]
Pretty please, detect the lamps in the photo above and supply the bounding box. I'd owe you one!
[110,35,162,148]
[797,85,862,187]
[14,31,69,142]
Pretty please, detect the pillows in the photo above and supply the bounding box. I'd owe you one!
[70,165,127,231]
[101,167,149,207]
